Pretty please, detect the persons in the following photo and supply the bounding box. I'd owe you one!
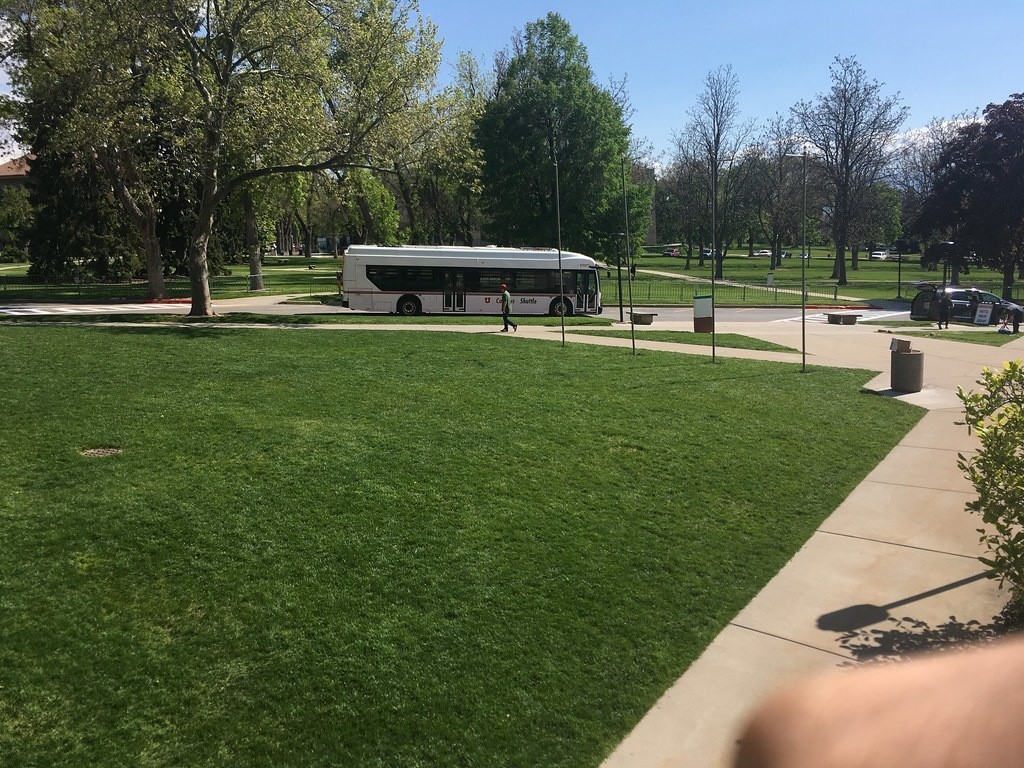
[501,284,517,332]
[938,292,954,329]
[336,270,343,297]
[607,264,611,279]
[999,306,1022,333]
[630,264,637,280]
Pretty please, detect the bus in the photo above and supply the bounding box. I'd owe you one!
[342,244,611,317]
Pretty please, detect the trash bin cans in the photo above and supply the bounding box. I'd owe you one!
[767,273,774,287]
[693,295,713,333]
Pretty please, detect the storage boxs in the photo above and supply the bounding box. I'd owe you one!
[889,338,910,353]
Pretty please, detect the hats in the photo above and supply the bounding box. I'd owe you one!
[498,284,507,290]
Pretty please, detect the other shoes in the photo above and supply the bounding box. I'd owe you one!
[939,325,942,329]
[501,329,508,332]
[514,325,517,332]
[946,327,948,329]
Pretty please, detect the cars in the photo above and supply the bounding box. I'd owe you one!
[780,251,793,260]
[796,251,813,260]
[844,237,1004,266]
[751,249,773,257]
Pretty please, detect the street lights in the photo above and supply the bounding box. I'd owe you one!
[706,147,734,363]
[785,145,808,375]
[620,156,643,355]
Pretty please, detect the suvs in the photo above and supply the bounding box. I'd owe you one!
[909,280,1024,324]
[702,248,716,260]
[663,248,681,257]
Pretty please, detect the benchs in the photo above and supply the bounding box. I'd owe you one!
[626,311,658,325]
[823,312,863,325]
